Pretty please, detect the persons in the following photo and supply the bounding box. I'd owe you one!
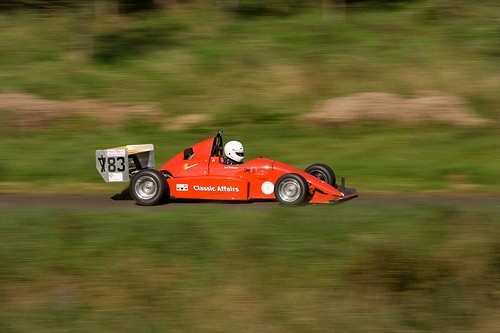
[221,140,247,165]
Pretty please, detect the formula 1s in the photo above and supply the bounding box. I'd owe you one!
[96,131,360,206]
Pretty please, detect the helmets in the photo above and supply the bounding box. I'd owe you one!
[224,140,246,162]
